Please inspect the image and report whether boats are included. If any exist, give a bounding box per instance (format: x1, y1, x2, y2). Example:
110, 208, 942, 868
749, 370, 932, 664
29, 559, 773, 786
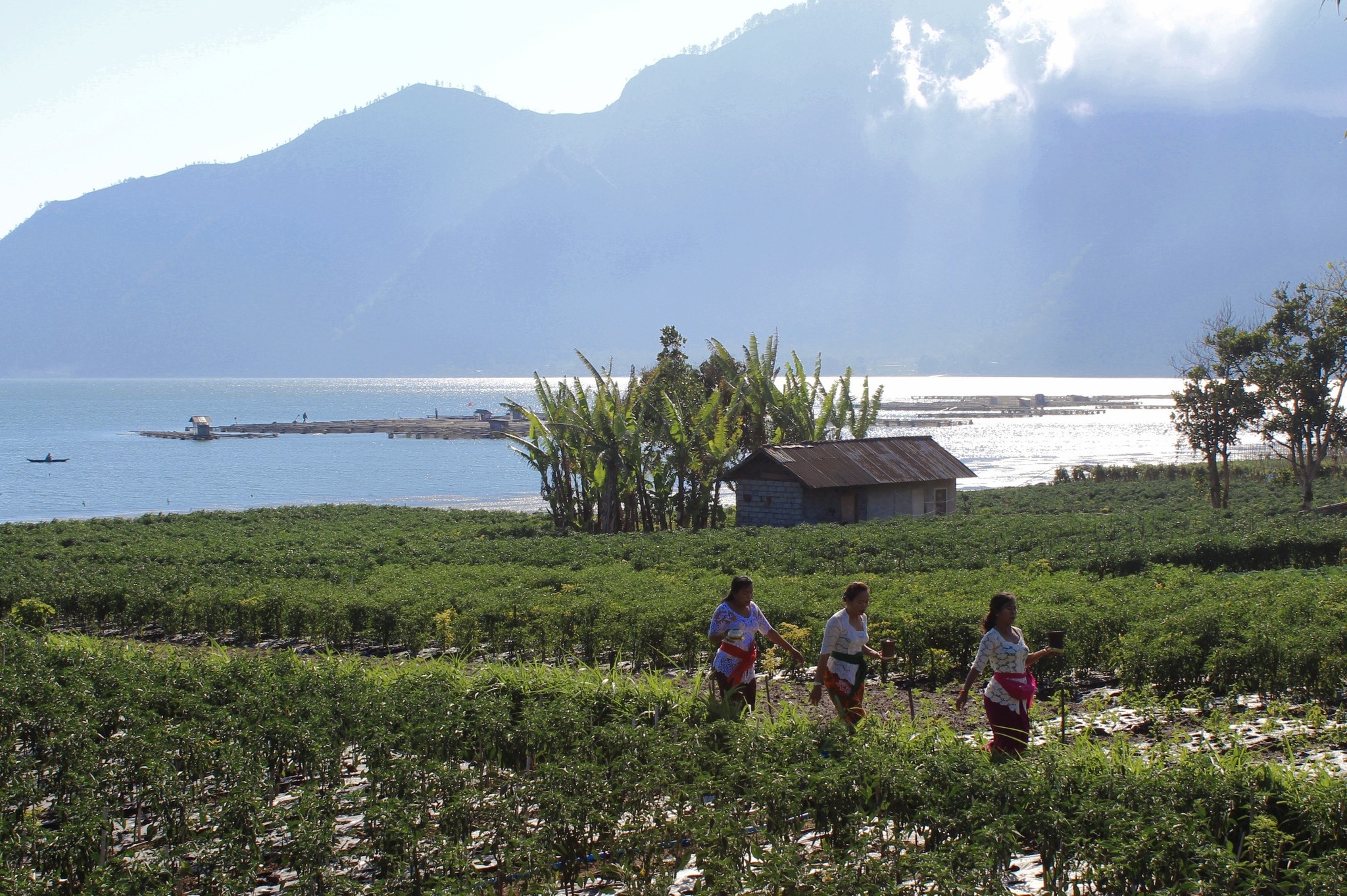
26, 458, 71, 462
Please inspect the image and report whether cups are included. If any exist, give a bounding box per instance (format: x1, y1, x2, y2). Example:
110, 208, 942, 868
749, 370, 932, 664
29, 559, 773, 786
880, 639, 897, 657
1046, 631, 1064, 649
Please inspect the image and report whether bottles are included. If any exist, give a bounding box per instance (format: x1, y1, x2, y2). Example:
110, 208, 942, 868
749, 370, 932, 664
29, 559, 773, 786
728, 628, 744, 640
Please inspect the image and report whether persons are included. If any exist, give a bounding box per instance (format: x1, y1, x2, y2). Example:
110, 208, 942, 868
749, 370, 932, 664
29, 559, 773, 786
303, 412, 308, 422
434, 408, 438, 418
45, 453, 54, 460
955, 592, 1057, 754
709, 575, 805, 713
808, 582, 893, 726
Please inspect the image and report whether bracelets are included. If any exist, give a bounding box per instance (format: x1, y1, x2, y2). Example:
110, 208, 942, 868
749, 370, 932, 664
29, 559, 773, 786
814, 682, 822, 686
959, 690, 968, 694
